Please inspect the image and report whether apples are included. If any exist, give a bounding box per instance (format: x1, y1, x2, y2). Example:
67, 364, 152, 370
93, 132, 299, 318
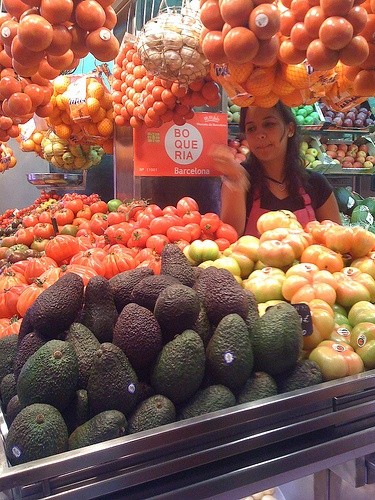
227, 138, 250, 163
320, 102, 375, 127
225, 95, 320, 125
296, 134, 323, 169
321, 142, 375, 168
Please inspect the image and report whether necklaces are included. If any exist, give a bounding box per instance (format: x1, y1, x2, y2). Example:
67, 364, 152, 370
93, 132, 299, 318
264, 174, 289, 192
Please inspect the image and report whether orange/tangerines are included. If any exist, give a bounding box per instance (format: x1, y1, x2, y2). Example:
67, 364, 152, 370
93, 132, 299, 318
0, 0, 120, 174
198, 0, 375, 98
112, 42, 221, 128
209, 58, 368, 112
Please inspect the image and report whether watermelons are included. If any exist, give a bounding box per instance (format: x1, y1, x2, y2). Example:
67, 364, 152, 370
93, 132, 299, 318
333, 186, 375, 234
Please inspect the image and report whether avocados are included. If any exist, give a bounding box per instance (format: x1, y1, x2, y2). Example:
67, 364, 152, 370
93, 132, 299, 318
0, 245, 323, 468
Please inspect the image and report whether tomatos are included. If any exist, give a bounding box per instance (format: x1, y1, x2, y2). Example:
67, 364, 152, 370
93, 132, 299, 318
0, 194, 238, 337
185, 211, 375, 379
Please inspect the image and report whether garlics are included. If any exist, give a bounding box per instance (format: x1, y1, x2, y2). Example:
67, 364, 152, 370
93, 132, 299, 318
136, 0, 209, 83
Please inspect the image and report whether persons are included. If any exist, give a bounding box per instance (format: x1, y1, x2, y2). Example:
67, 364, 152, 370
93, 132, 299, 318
207, 100, 343, 241
